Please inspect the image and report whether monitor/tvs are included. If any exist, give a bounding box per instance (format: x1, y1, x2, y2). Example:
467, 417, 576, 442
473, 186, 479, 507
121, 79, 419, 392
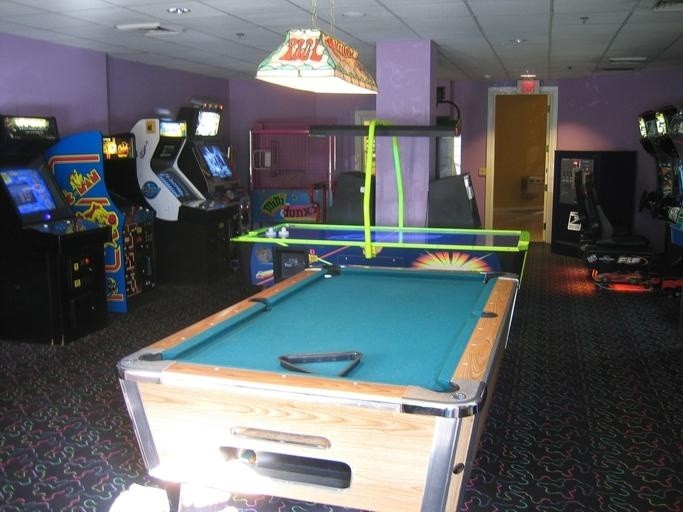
659, 161, 674, 199
197, 143, 234, 179
2, 166, 57, 215
158, 172, 193, 202
674, 157, 683, 203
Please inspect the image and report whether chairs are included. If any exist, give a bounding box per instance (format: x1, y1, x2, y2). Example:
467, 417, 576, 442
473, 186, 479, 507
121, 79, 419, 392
582, 158, 653, 285
573, 158, 652, 247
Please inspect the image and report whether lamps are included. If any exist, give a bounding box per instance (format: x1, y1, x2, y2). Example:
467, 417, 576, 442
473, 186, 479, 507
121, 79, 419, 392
253, 1, 383, 97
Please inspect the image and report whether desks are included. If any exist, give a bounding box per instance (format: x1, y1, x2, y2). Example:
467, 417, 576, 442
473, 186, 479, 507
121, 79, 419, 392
113, 261, 521, 512
